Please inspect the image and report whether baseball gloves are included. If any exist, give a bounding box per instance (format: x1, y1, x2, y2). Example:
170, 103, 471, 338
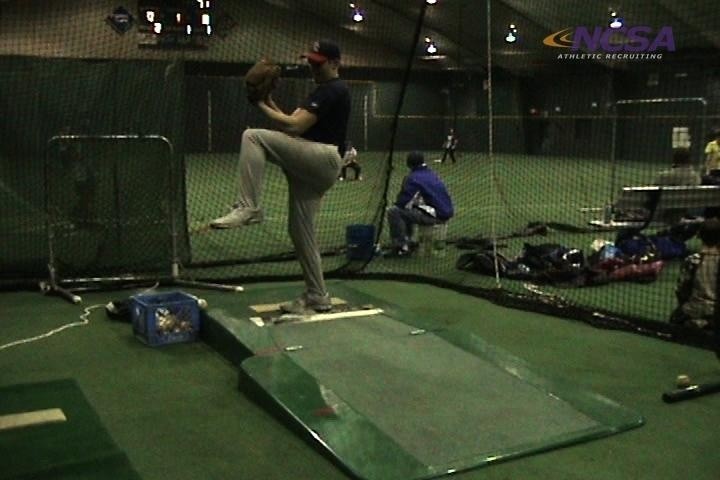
241, 57, 274, 104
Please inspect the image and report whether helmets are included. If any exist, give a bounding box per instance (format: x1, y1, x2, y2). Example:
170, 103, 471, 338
407, 151, 424, 166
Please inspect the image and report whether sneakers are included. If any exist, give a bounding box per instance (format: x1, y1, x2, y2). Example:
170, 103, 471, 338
208, 205, 264, 232
279, 292, 332, 313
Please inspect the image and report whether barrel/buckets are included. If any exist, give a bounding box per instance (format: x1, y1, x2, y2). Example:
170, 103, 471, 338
418, 222, 450, 260
345, 225, 374, 260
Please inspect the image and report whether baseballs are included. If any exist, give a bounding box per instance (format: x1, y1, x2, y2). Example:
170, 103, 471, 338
675, 374, 691, 388
196, 297, 209, 309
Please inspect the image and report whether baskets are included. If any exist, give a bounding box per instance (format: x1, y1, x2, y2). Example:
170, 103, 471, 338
128, 290, 200, 347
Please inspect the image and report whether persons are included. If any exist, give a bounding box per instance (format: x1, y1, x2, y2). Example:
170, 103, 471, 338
674, 218, 720, 328
653, 146, 703, 188
705, 126, 720, 185
208, 36, 352, 312
383, 151, 454, 258
440, 127, 459, 165
336, 140, 363, 181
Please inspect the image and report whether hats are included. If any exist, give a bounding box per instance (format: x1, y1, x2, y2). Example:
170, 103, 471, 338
299, 40, 341, 63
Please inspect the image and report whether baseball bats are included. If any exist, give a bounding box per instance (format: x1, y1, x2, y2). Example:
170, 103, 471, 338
154, 306, 193, 342
662, 381, 720, 402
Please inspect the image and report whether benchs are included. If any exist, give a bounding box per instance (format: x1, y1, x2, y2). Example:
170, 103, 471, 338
576, 183, 720, 241
